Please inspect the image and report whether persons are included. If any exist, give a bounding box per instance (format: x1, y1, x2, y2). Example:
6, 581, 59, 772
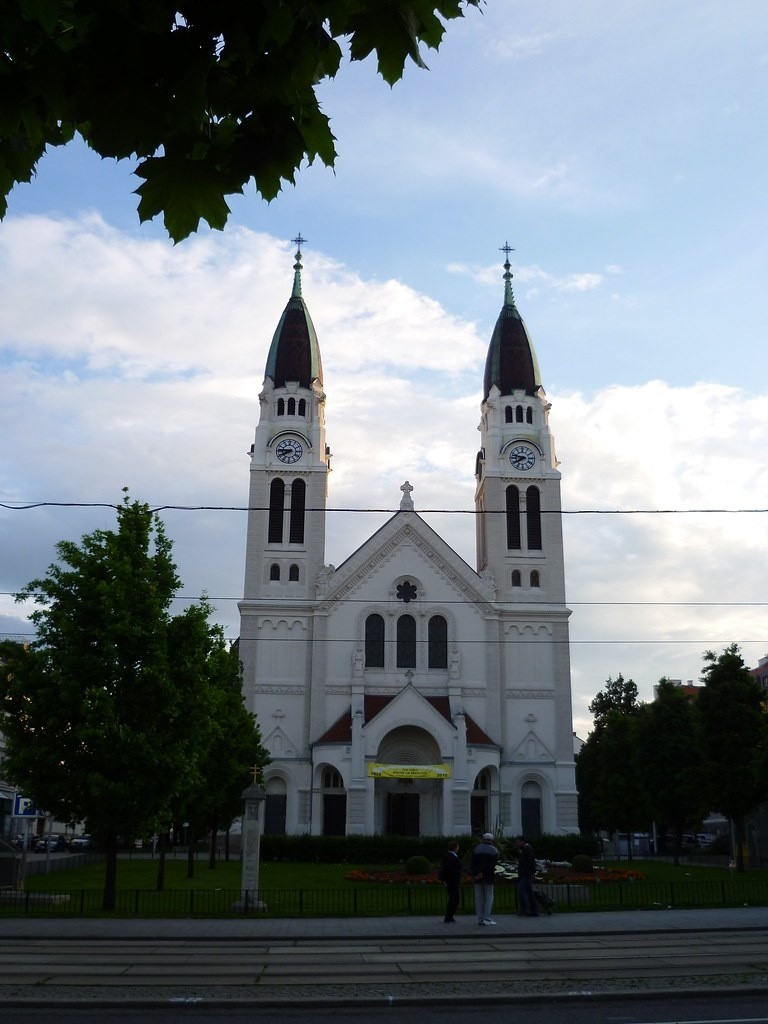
514, 836, 539, 917
440, 841, 463, 924
469, 833, 498, 925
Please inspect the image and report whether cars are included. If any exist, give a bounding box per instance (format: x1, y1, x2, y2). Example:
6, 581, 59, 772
35, 835, 66, 853
650, 834, 707, 855
69, 834, 93, 851
64, 834, 78, 846
12, 833, 39, 848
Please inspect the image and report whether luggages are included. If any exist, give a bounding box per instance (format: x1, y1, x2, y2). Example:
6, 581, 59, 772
533, 888, 556, 914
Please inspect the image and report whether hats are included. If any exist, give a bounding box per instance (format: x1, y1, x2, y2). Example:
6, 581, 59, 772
484, 832, 493, 839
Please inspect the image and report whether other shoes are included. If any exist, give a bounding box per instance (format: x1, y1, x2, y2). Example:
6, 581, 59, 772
517, 912, 527, 915
443, 918, 456, 924
479, 920, 484, 926
483, 919, 497, 925
526, 912, 540, 917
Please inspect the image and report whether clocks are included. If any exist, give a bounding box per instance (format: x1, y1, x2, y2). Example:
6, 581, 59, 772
508, 446, 535, 471
276, 439, 303, 465
